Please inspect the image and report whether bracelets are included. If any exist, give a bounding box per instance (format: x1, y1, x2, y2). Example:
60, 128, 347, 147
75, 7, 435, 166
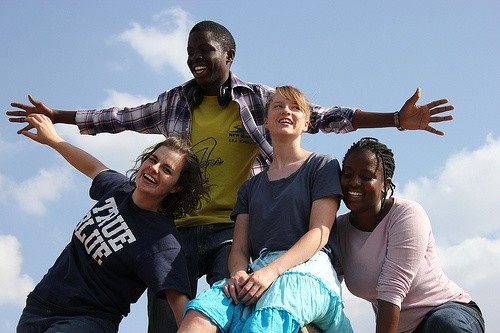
394, 111, 405, 132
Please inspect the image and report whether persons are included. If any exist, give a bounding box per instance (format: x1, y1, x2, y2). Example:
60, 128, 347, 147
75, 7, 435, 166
16, 114, 204, 333
175, 86, 353, 333
327, 137, 485, 333
5, 20, 455, 333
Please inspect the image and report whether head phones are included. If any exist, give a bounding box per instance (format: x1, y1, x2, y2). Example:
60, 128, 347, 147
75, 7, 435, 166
186, 70, 232, 107
